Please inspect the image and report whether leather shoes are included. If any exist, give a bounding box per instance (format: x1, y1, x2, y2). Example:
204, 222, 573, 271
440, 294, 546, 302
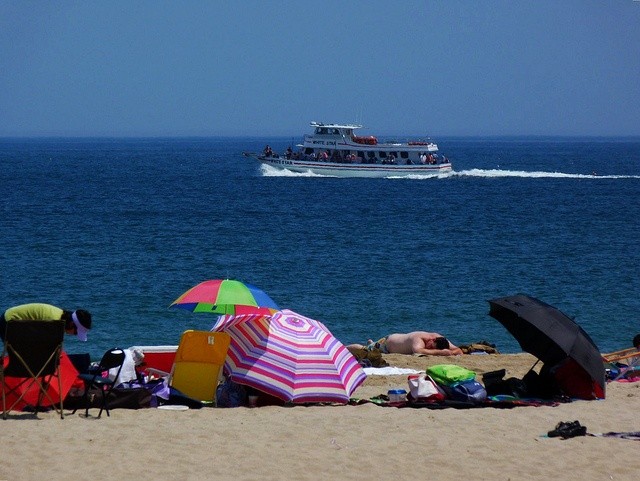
547, 421, 581, 438
556, 424, 587, 438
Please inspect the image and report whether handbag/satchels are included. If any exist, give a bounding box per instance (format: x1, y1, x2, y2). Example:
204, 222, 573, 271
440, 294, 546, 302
215, 376, 249, 407
407, 372, 444, 402
67, 387, 151, 410
119, 376, 170, 408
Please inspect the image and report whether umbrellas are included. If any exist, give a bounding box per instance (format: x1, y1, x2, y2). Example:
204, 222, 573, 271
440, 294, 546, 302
486, 291, 607, 402
167, 268, 283, 321
209, 309, 368, 405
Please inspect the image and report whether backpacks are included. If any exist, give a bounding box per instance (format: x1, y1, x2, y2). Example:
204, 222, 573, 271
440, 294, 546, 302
438, 377, 487, 403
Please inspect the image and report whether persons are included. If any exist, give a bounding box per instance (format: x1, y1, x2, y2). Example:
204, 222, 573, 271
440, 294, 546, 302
258, 143, 278, 163
286, 145, 329, 162
0, 302, 95, 345
416, 152, 447, 164
344, 152, 356, 164
384, 155, 395, 163
342, 330, 464, 358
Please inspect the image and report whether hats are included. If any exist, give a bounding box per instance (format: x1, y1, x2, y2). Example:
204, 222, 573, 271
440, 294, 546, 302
72, 308, 91, 341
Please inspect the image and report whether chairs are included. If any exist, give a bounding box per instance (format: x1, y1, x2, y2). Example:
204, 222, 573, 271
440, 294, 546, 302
146, 329, 231, 407
1, 320, 66, 419
71, 346, 125, 419
67, 353, 91, 374
136, 352, 175, 377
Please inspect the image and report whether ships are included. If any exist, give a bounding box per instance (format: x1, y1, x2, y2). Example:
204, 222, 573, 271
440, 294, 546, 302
243, 121, 452, 178
